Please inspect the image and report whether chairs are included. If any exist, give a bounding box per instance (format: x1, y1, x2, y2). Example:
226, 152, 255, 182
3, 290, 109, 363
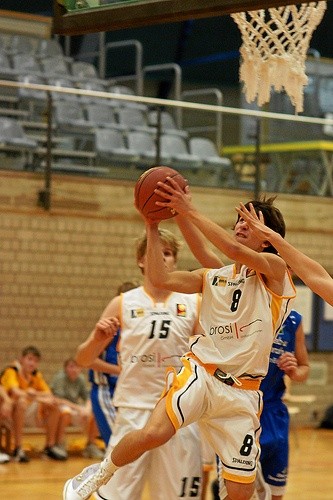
0, 32, 231, 186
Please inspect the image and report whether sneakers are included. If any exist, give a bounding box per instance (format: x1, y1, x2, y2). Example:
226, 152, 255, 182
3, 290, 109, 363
63, 464, 113, 500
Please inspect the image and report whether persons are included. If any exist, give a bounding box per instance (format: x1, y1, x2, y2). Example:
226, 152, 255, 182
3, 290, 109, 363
0, 345, 107, 464
63, 176, 296, 500
236, 202, 333, 307
216, 309, 311, 500
73, 227, 216, 500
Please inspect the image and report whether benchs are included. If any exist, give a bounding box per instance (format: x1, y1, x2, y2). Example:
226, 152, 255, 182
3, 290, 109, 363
1, 423, 107, 455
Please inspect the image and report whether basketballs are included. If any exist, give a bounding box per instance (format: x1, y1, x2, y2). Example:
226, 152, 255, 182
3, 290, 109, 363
134, 166, 188, 221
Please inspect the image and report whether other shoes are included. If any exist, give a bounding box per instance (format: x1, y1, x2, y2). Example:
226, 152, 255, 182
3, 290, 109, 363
44, 446, 67, 461
83, 445, 103, 459
0, 452, 10, 463
14, 449, 28, 461
